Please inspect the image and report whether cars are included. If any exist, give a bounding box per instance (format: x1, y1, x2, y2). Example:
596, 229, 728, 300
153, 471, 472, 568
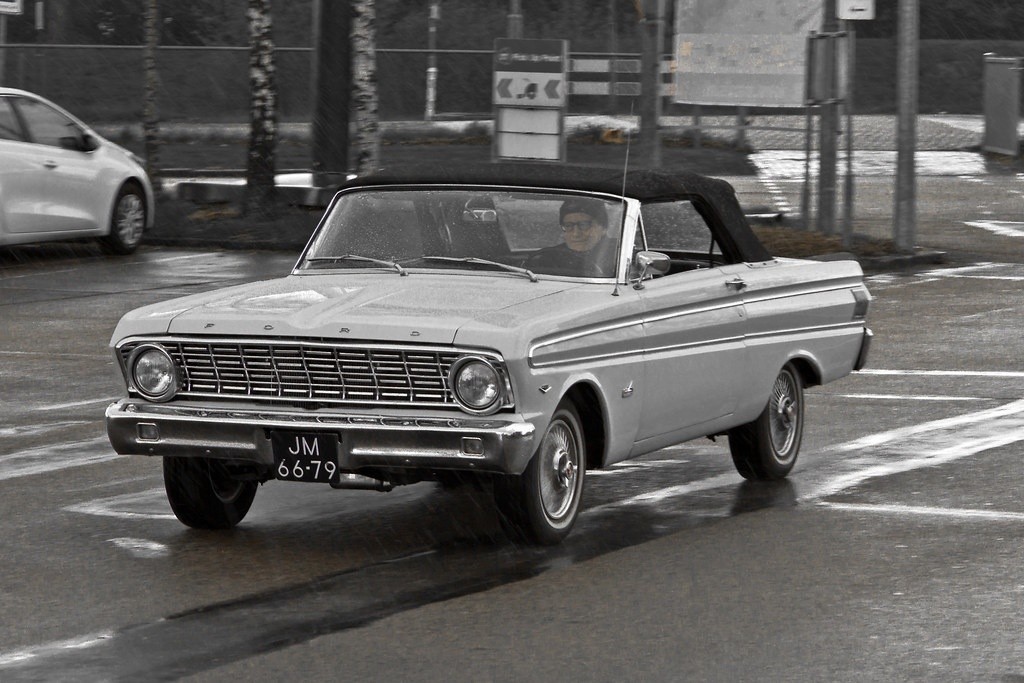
100, 155, 875, 549
0, 85, 157, 256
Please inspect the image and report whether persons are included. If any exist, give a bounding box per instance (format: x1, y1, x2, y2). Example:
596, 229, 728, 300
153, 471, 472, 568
519, 198, 618, 277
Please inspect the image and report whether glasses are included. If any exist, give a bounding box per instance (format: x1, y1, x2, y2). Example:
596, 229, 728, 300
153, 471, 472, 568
559, 221, 595, 233
